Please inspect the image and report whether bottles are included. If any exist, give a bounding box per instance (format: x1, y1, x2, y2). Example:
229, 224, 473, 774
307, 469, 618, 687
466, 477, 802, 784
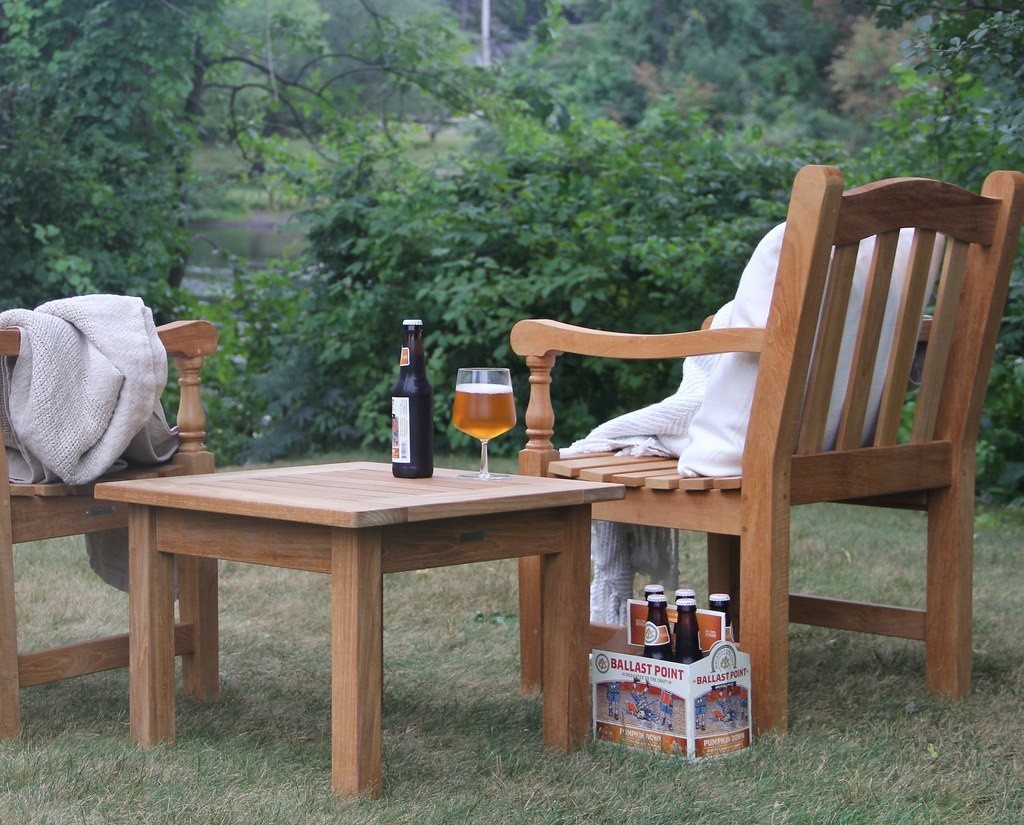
710, 593, 735, 643
392, 319, 434, 479
644, 584, 664, 603
674, 590, 696, 607
641, 594, 673, 661
672, 599, 703, 665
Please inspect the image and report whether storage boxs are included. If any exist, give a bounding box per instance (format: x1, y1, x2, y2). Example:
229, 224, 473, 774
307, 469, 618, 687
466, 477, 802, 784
590, 598, 753, 763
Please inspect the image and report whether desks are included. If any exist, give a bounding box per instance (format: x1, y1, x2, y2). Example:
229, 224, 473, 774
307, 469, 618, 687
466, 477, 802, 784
94, 460, 626, 798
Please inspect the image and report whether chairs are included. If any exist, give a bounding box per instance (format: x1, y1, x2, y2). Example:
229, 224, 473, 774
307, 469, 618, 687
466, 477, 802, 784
509, 164, 1024, 739
0, 293, 220, 737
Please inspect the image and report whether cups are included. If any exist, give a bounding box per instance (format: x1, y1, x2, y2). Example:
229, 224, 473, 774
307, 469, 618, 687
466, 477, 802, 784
452, 368, 516, 480
